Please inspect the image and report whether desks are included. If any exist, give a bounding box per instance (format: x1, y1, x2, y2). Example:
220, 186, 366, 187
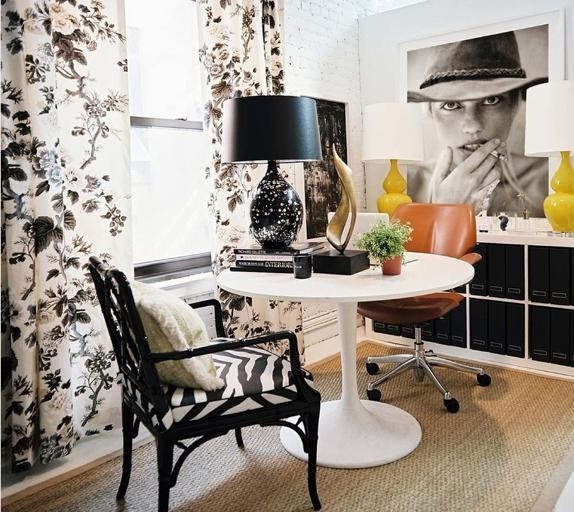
215, 252, 476, 470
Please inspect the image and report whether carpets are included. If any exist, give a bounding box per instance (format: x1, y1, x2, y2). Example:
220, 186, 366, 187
1, 340, 574, 512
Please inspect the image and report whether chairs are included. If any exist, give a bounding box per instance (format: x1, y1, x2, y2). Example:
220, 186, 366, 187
88, 256, 322, 511
356, 203, 491, 413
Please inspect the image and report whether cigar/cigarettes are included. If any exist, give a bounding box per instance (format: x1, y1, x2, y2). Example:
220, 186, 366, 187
477, 143, 510, 163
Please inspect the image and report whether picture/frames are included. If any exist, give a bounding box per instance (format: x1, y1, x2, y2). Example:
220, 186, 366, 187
295, 93, 349, 242
396, 9, 566, 231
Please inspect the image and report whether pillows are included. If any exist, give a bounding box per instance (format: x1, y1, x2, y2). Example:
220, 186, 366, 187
128, 277, 227, 393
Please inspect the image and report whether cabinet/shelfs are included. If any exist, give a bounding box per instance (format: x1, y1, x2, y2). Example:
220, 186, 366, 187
364, 231, 574, 384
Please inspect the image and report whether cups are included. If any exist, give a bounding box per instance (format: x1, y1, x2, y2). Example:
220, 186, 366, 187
292, 253, 311, 280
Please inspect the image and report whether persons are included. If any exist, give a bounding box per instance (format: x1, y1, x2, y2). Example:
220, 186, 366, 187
404, 32, 549, 219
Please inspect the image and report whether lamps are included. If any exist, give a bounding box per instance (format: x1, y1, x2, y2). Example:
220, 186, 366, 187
221, 95, 324, 253
524, 81, 574, 233
362, 101, 425, 221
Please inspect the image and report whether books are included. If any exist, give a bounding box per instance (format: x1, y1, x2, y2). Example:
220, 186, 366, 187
228, 241, 329, 274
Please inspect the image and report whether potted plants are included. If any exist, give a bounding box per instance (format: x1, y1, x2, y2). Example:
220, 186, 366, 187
353, 219, 414, 275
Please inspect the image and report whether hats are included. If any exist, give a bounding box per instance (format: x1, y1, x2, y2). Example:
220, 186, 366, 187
408, 31, 548, 108
